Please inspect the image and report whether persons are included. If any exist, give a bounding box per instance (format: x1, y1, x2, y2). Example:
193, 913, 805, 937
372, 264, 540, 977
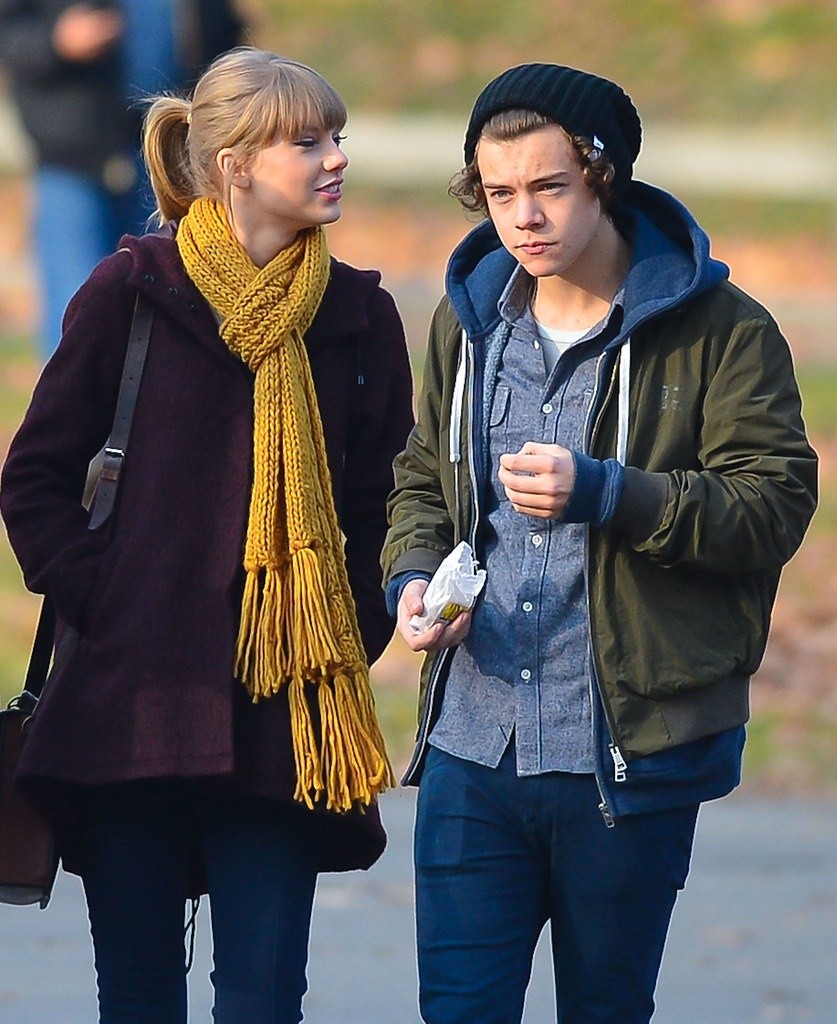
1, 0, 254, 367
378, 64, 818, 1024
0, 52, 412, 1024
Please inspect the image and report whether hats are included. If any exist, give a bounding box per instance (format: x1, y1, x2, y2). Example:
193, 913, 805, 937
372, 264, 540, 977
464, 62, 641, 194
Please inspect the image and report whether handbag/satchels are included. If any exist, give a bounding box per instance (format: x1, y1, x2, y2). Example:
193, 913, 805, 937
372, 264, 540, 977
0, 690, 61, 911
